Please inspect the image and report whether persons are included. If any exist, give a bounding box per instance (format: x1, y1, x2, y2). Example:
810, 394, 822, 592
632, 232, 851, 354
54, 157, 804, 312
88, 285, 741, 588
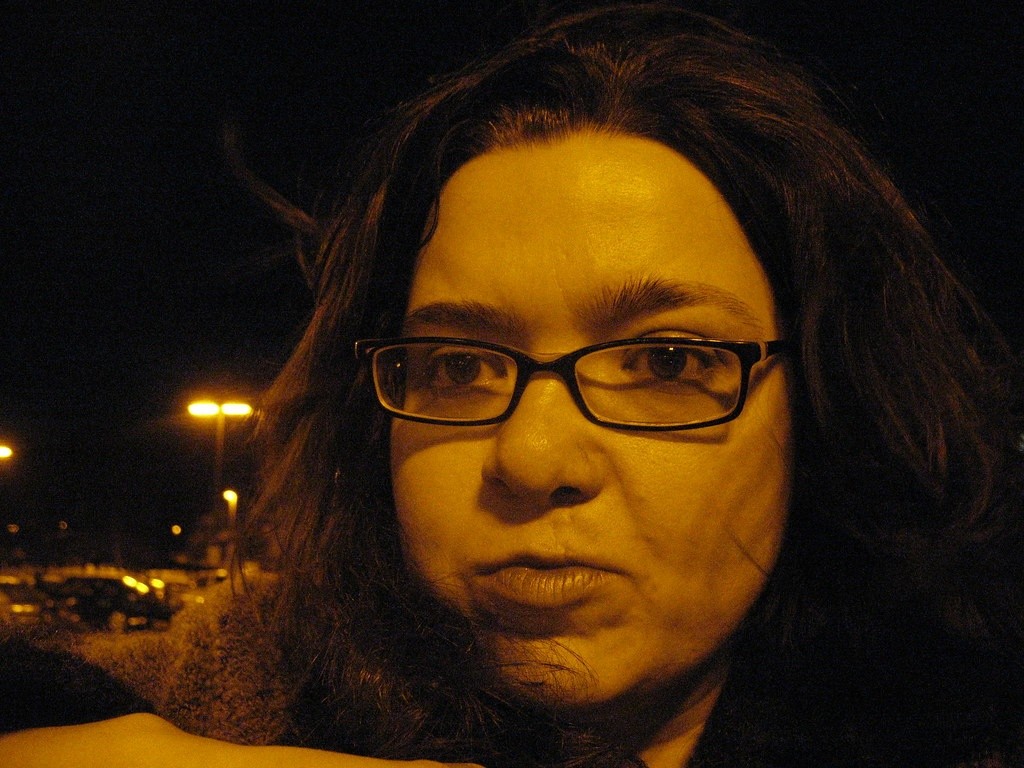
0, 1, 1024, 768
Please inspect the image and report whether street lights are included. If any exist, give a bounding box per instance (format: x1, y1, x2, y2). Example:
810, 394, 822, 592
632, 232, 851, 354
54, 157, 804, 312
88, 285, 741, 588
185, 401, 253, 520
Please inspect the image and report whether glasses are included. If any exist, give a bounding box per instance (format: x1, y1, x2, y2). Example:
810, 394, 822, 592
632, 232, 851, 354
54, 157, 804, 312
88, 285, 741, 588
355, 338, 791, 431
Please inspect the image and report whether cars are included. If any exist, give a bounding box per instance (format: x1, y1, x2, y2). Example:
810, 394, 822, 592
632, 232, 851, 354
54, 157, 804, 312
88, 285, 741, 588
35, 569, 185, 633
